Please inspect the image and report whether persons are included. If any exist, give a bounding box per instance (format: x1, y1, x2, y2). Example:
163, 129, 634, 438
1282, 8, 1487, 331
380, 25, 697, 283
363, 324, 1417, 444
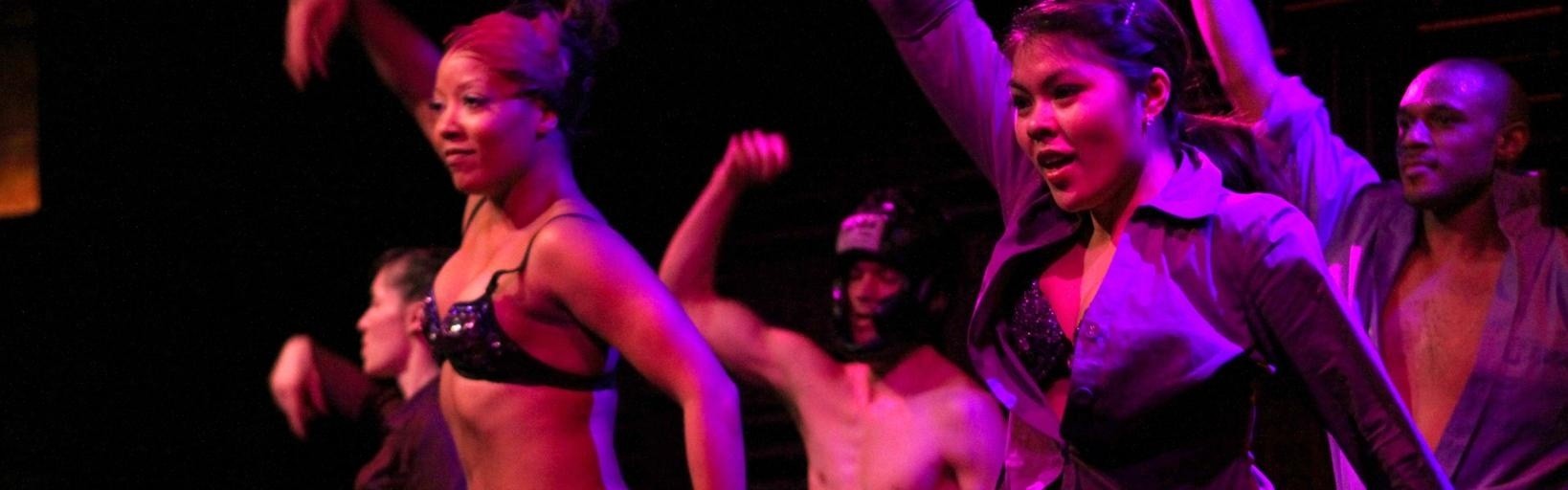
655, 126, 1006, 490
1189, 0, 1567, 488
1175, 58, 1261, 193
866, 2, 1459, 490
281, 0, 751, 490
266, 244, 471, 490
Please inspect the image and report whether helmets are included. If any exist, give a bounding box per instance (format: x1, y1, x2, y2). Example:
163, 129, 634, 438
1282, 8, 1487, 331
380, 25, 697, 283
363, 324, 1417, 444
829, 206, 955, 341
